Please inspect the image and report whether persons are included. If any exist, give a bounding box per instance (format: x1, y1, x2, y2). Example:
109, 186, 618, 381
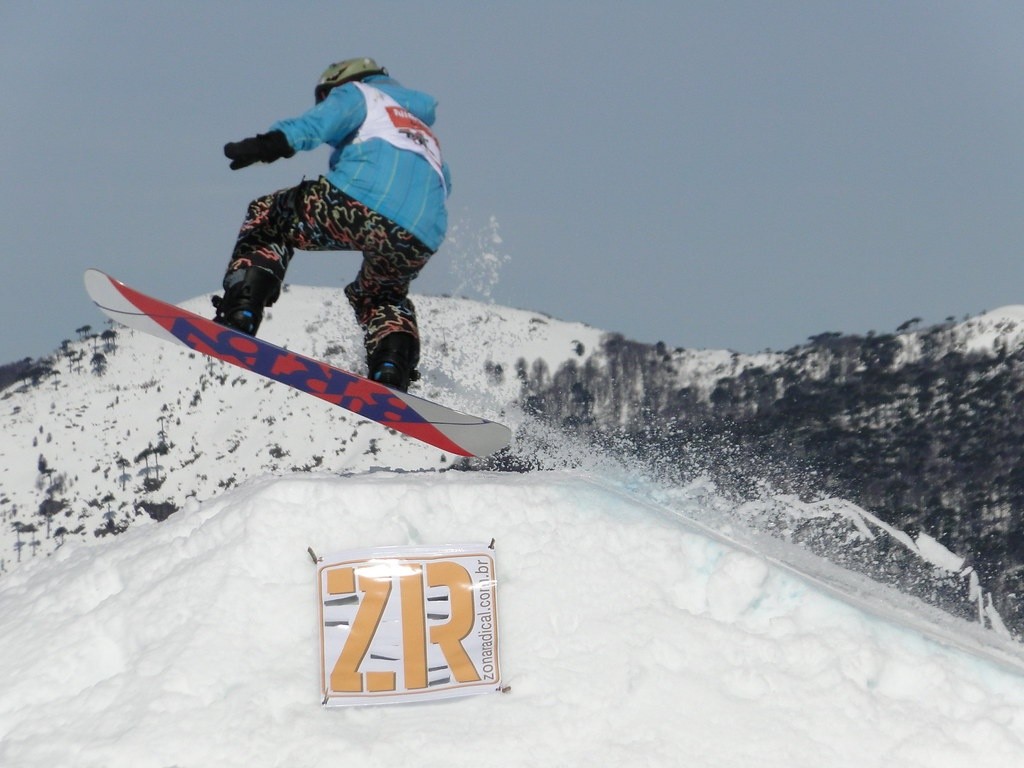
198, 55, 451, 393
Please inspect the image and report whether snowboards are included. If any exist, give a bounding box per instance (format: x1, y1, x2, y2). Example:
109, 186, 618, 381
81, 267, 514, 461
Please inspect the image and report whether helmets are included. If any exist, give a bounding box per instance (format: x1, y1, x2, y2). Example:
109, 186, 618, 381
315, 57, 382, 107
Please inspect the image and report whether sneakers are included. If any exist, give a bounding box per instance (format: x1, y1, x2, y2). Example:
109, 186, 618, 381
213, 284, 263, 334
366, 350, 421, 396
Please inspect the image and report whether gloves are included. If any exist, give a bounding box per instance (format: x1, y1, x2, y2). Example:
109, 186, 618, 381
224, 131, 295, 169
